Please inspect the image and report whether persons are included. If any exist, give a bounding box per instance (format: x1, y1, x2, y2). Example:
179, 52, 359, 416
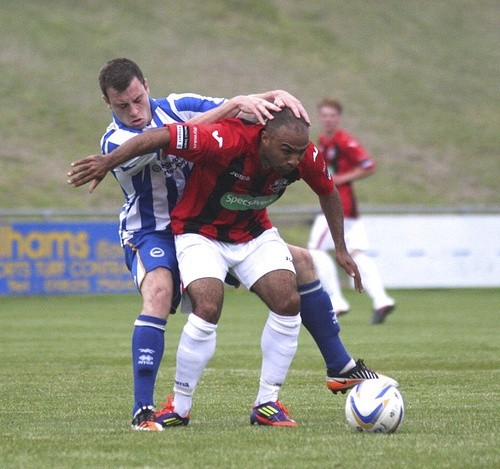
96, 57, 400, 430
67, 104, 364, 426
307, 99, 395, 324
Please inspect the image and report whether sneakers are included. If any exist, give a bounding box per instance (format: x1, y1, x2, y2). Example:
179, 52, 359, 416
130, 401, 164, 430
251, 400, 297, 427
152, 396, 189, 426
326, 358, 399, 394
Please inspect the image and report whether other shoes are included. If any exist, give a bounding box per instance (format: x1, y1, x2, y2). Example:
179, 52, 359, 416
371, 305, 395, 323
332, 303, 349, 315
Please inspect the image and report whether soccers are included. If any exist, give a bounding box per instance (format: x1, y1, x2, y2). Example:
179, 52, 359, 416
345, 378, 404, 434
